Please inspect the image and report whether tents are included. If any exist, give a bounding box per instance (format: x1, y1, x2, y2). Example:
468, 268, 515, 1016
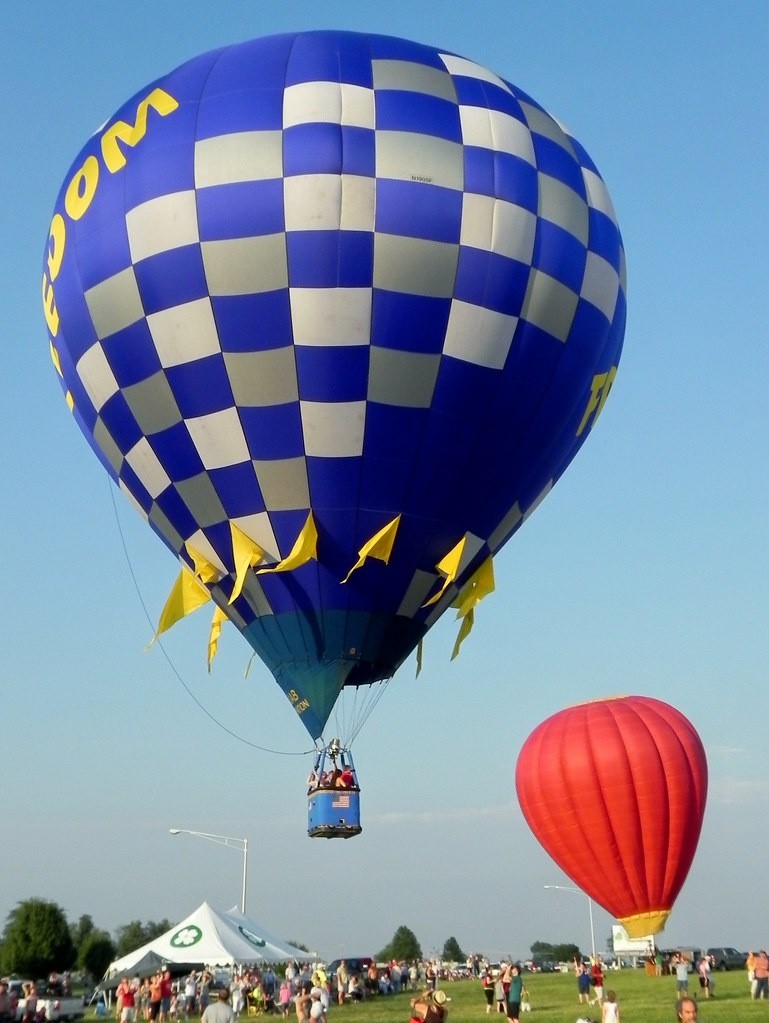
86, 902, 318, 1010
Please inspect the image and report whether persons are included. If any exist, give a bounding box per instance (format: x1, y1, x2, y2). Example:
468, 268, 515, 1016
576, 956, 607, 1007
602, 990, 619, 1023
482, 954, 531, 1023
698, 955, 715, 997
466, 956, 490, 979
676, 997, 697, 1023
116, 961, 330, 1023
746, 950, 769, 999
0, 972, 72, 1023
654, 952, 662, 976
307, 764, 355, 788
672, 956, 689, 999
337, 959, 454, 1005
409, 991, 448, 1023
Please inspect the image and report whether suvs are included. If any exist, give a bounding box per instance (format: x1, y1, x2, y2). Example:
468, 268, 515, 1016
705, 946, 748, 971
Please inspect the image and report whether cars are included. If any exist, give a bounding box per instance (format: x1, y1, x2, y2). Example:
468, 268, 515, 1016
489, 963, 503, 976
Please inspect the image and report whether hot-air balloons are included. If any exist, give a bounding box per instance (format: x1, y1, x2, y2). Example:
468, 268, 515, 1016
511, 693, 710, 978
36, 27, 629, 843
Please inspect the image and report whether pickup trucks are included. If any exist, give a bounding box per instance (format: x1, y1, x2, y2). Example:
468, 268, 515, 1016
7, 978, 86, 1023
326, 955, 391, 991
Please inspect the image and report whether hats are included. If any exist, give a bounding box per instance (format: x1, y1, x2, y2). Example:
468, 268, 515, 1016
310, 1001, 323, 1019
432, 990, 446, 1008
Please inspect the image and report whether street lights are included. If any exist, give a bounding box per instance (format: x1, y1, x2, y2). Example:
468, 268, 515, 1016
543, 884, 596, 963
169, 828, 248, 915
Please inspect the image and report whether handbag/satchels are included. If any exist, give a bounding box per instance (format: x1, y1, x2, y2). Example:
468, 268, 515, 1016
590, 977, 597, 986
